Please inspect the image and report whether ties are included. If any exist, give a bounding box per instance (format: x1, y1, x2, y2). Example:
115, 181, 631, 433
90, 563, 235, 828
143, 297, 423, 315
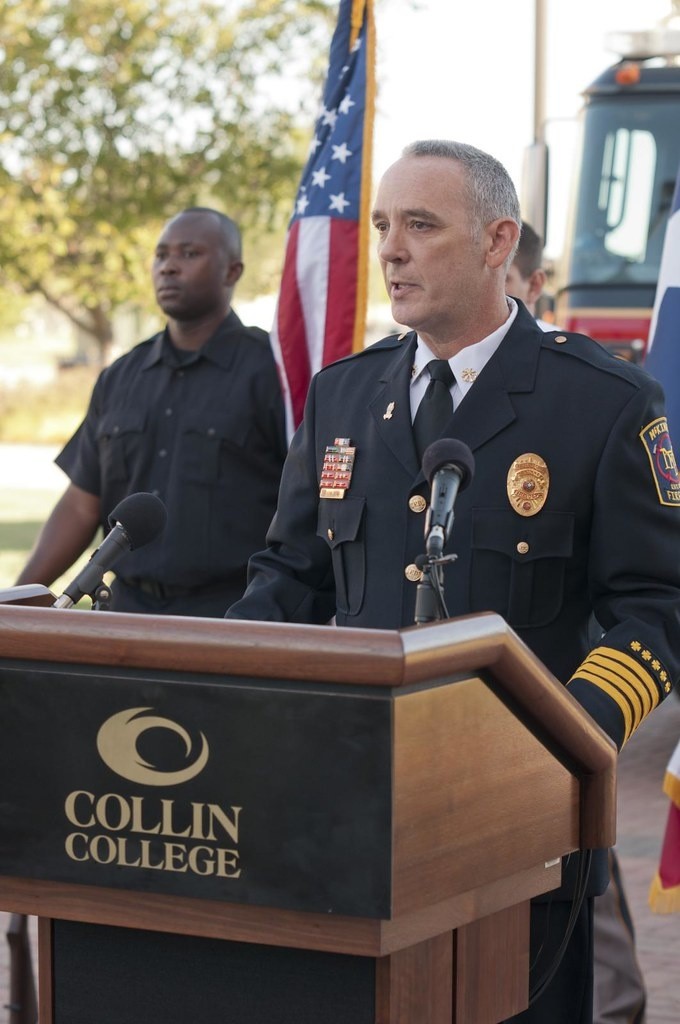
412, 360, 457, 467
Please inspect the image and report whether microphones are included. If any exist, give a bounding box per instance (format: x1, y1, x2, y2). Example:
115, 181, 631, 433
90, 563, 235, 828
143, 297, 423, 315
51, 492, 168, 608
421, 439, 477, 569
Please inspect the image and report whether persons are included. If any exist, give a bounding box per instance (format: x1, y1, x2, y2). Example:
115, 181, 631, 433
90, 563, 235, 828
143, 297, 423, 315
219, 140, 680, 1024
11, 201, 295, 623
502, 219, 562, 338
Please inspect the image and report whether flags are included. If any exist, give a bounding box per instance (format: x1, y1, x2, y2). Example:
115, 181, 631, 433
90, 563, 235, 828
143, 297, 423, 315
635, 153, 680, 919
257, 0, 383, 463
